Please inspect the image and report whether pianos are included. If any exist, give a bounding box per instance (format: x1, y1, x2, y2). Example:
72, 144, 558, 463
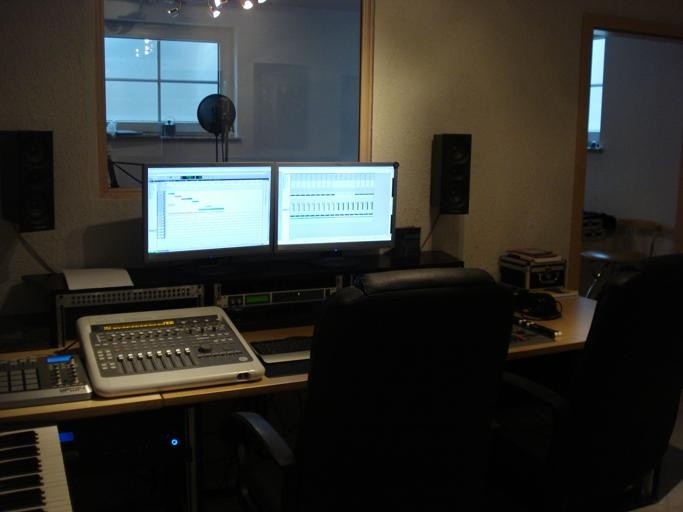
0, 425, 73, 512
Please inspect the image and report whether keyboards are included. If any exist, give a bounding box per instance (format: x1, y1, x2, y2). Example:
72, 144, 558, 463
248, 336, 313, 363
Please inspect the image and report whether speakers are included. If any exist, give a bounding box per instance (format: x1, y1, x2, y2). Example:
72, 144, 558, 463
428, 131, 472, 216
0, 130, 57, 232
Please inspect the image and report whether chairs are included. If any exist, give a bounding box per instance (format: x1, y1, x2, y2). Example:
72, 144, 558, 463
581, 218, 665, 297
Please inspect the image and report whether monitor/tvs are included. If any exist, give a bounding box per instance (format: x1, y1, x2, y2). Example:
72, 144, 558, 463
273, 160, 399, 268
141, 162, 274, 276
